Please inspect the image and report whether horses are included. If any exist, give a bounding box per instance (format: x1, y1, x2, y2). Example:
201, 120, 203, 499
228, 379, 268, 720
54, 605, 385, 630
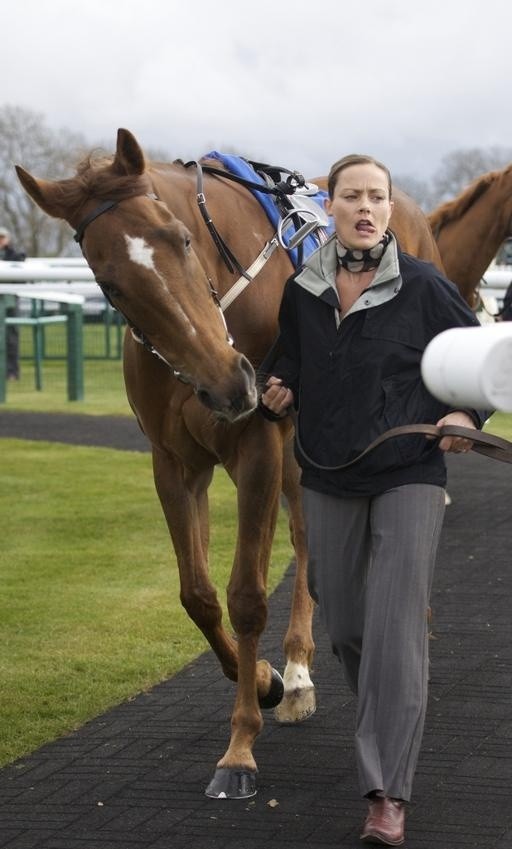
426, 159, 512, 300
13, 123, 451, 806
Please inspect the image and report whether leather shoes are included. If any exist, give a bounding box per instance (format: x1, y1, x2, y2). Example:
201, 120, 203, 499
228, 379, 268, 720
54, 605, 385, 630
360, 797, 406, 846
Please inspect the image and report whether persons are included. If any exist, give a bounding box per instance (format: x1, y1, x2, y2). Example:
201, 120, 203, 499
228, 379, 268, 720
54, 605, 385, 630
0, 229, 26, 381
263, 155, 498, 847
